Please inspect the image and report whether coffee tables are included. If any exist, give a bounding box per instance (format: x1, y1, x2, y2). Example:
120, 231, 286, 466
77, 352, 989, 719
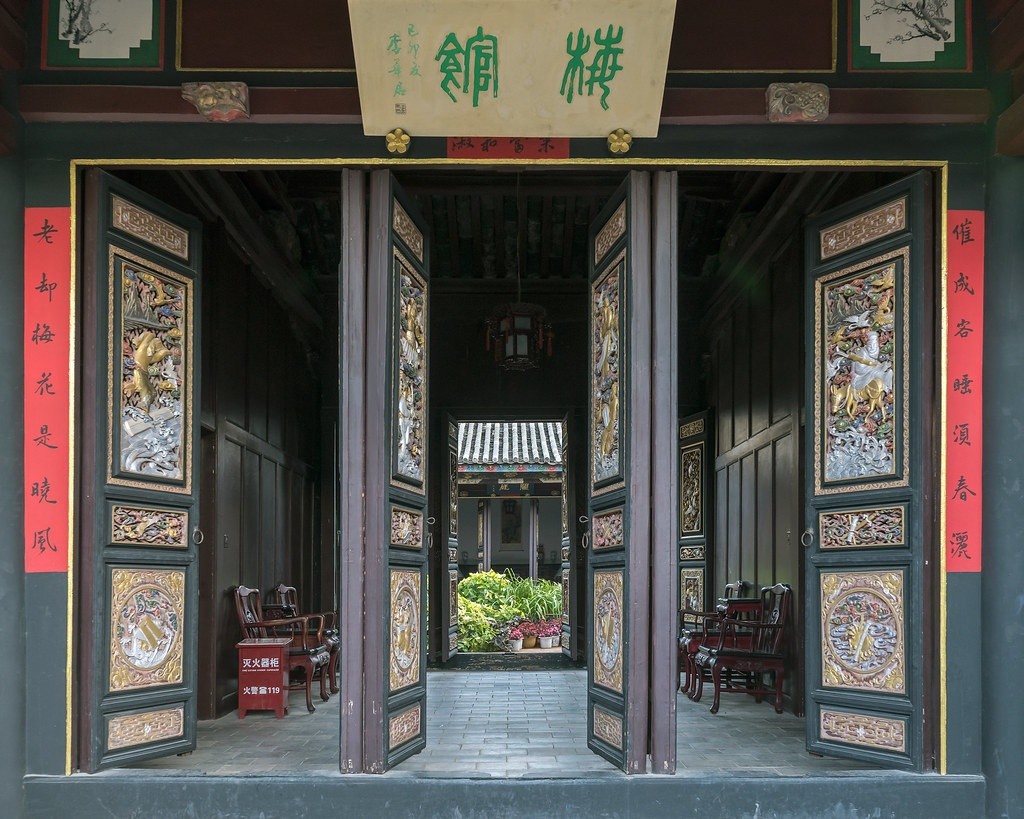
267, 629, 339, 651
681, 628, 759, 700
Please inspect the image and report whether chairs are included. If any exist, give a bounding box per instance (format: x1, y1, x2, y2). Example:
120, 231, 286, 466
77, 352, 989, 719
679, 578, 793, 714
233, 586, 340, 715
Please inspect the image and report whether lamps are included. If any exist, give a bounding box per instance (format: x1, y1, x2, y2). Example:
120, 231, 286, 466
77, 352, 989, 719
486, 173, 553, 370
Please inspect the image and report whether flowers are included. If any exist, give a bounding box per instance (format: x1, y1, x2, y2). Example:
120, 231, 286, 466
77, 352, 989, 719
507, 619, 563, 639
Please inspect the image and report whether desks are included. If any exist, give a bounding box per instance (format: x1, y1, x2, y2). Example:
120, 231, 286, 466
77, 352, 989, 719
716, 597, 764, 629
262, 603, 296, 629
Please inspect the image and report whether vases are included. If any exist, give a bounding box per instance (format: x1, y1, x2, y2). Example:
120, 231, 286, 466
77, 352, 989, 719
508, 634, 561, 652
